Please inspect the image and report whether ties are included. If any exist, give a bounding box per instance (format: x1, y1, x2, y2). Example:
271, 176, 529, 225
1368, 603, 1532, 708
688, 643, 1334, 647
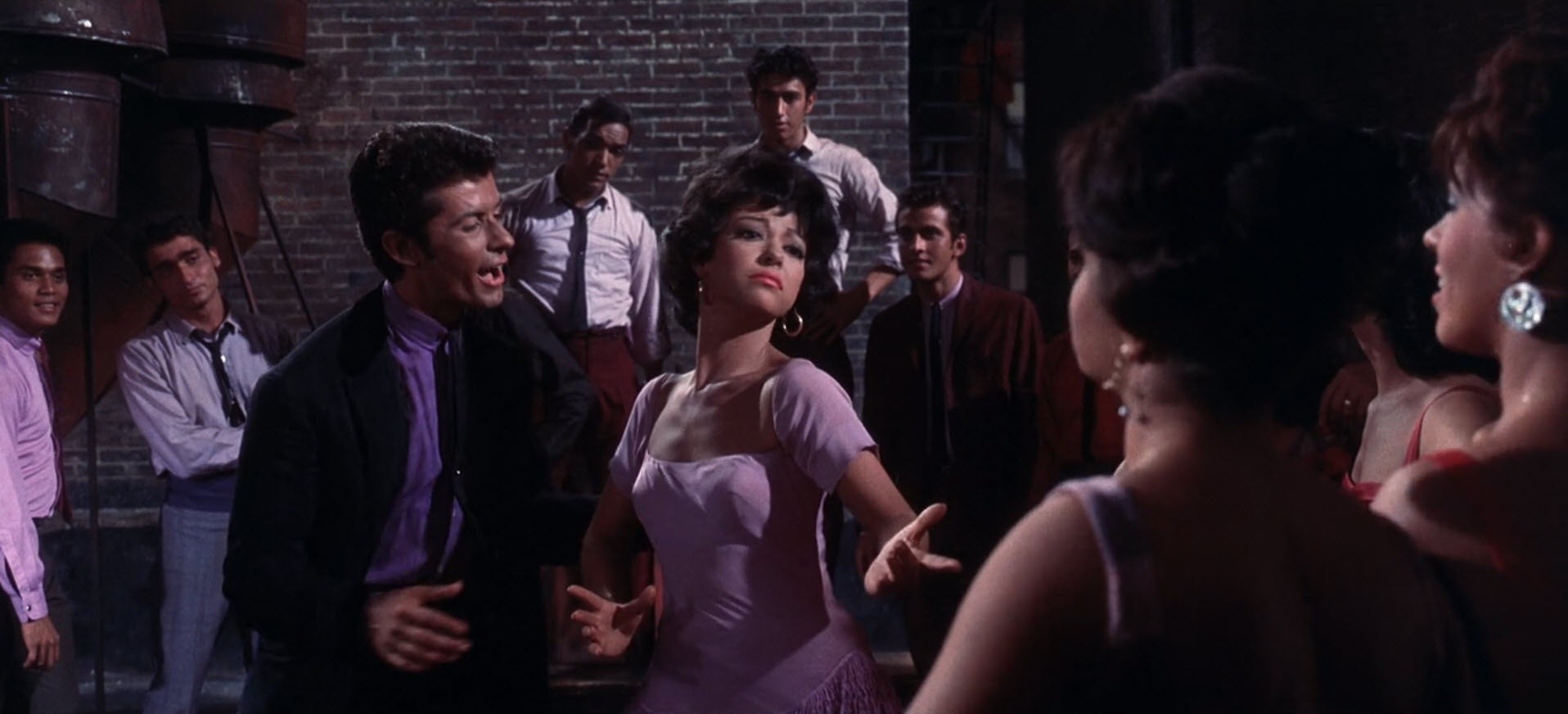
930, 303, 942, 469
39, 343, 74, 524
559, 201, 603, 332
191, 326, 247, 426
423, 332, 462, 584
1081, 380, 1097, 479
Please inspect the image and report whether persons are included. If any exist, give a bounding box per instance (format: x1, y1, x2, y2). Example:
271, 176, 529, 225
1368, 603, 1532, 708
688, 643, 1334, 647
1030, 227, 1124, 504
659, 43, 905, 578
0, 217, 73, 714
1368, 20, 1568, 714
905, 65, 1480, 714
861, 182, 1045, 667
115, 211, 293, 714
566, 145, 961, 714
500, 97, 671, 671
221, 121, 579, 714
499, 289, 590, 464
1338, 128, 1501, 509
